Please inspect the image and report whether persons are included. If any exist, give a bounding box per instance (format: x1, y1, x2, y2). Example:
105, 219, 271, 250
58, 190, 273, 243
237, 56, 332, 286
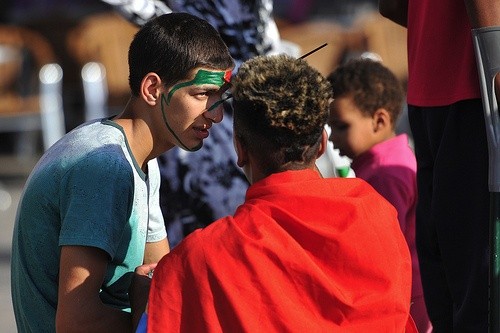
147, 53, 416, 333
11, 12, 236, 333
327, 59, 432, 332
379, 0, 500, 333
